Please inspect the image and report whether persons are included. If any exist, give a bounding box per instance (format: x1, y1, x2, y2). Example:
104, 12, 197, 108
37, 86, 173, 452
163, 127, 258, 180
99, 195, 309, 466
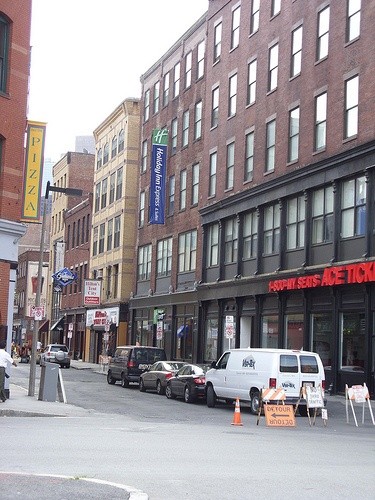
11, 339, 42, 359
0, 340, 18, 403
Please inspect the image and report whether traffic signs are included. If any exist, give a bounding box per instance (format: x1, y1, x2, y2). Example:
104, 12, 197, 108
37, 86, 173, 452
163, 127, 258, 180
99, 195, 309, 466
263, 404, 294, 428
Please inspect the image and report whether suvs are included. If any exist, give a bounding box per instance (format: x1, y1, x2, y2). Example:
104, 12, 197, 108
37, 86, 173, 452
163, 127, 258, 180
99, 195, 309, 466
40, 344, 69, 369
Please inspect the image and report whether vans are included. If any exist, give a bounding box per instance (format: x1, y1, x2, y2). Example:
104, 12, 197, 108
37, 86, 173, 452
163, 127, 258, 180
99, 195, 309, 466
206, 348, 326, 417
106, 346, 166, 388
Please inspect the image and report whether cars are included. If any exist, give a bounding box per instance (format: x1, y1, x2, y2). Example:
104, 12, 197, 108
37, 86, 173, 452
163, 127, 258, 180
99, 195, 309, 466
165, 364, 211, 404
138, 360, 186, 393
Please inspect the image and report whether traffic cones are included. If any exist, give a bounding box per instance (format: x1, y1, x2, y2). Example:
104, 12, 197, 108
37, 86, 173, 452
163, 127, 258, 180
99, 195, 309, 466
231, 397, 242, 425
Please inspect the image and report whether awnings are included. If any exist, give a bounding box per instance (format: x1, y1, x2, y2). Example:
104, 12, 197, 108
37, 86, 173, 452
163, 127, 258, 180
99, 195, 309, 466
178, 325, 191, 336
39, 315, 66, 332
